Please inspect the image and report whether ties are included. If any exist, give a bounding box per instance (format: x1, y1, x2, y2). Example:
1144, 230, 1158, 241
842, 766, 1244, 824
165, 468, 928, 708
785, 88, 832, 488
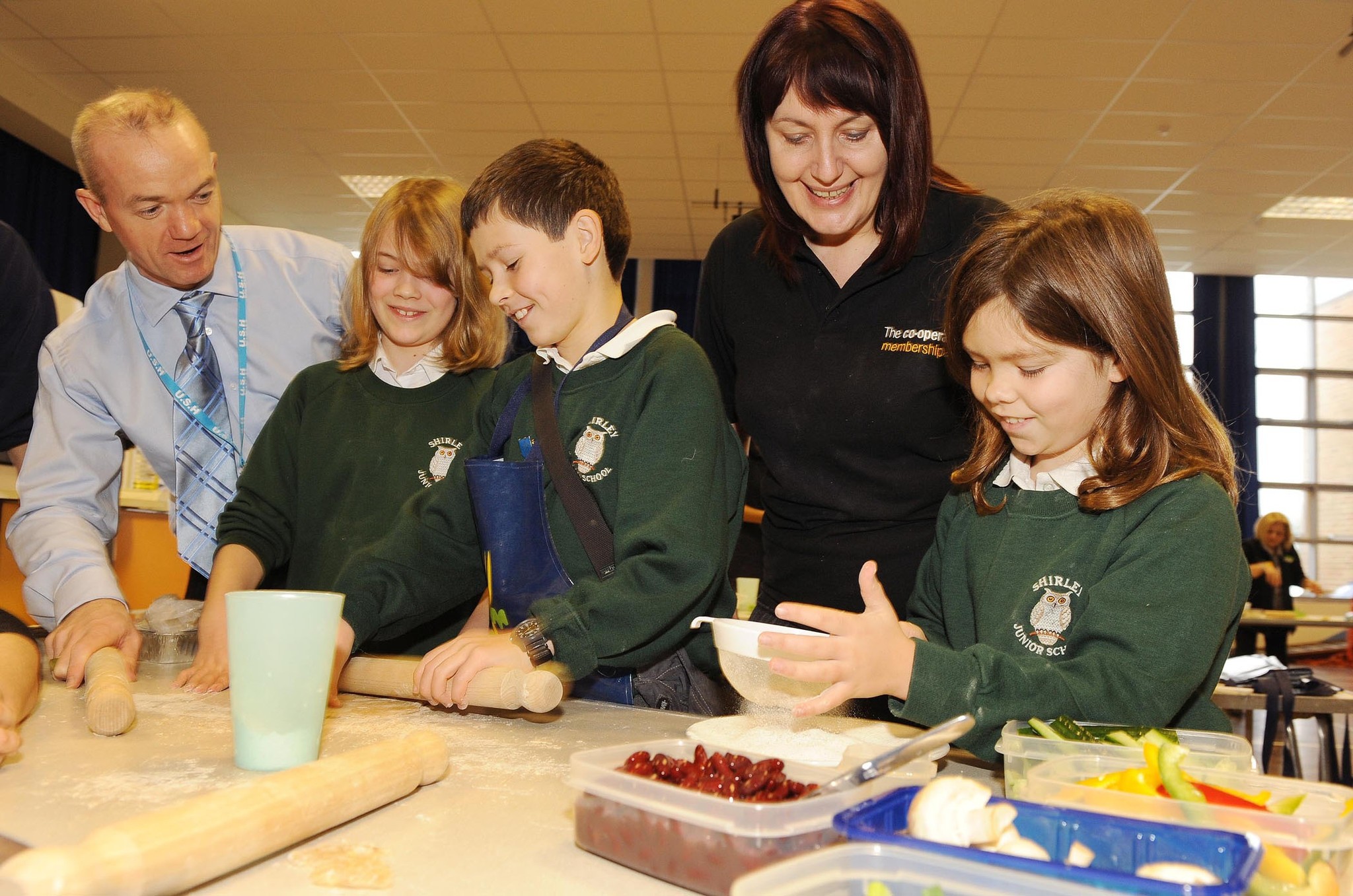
173, 290, 238, 580
1273, 555, 1283, 610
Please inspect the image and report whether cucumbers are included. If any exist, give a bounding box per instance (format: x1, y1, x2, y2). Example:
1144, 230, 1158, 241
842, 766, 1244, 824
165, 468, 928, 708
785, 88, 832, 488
1027, 715, 1180, 746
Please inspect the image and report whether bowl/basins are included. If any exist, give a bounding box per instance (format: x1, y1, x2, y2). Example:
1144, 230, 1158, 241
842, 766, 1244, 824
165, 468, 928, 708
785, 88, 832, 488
137, 628, 198, 664
689, 616, 834, 708
567, 713, 1352, 896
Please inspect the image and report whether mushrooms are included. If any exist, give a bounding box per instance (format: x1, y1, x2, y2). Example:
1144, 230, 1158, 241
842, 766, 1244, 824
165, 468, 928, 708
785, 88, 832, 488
895, 775, 1224, 885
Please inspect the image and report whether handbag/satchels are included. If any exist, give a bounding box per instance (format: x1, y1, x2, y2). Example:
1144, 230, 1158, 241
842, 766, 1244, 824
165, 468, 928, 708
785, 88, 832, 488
634, 665, 739, 717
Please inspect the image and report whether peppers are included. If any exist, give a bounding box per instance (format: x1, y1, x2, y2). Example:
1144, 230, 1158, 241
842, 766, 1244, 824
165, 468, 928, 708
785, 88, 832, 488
1080, 741, 1353, 895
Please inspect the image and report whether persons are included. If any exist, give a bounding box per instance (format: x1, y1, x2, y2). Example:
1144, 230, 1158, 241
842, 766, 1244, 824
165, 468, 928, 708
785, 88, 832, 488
1, 86, 750, 752
759, 188, 1251, 765
692, 0, 1017, 735
1240, 512, 1329, 666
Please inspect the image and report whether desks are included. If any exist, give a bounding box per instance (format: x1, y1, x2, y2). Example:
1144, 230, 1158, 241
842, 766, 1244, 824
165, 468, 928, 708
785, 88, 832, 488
1208, 676, 1353, 785
0, 446, 192, 627
1238, 608, 1353, 628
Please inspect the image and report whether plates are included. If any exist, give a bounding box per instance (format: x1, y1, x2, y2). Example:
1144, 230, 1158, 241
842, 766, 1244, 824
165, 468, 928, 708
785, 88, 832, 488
686, 714, 951, 762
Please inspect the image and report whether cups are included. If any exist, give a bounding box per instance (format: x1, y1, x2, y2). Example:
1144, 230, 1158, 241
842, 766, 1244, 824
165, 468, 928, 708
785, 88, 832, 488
225, 589, 346, 771
